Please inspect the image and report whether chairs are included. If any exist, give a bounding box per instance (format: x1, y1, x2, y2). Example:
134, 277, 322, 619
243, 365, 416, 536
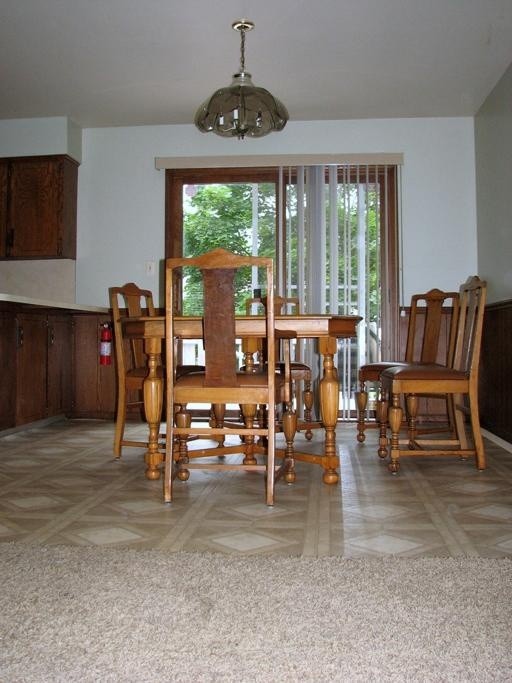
243, 295, 313, 440
165, 248, 284, 506
107, 283, 178, 459
379, 271, 488, 474
355, 287, 460, 443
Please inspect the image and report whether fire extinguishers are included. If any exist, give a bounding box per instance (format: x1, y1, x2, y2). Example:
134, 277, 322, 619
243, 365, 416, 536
99, 321, 112, 365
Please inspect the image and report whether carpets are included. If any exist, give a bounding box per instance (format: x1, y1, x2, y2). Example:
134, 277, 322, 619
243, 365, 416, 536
0, 543, 512, 681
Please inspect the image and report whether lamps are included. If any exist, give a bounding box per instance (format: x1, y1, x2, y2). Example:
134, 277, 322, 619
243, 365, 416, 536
193, 20, 288, 139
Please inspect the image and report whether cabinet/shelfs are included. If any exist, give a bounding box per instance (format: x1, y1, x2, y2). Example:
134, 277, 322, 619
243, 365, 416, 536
18, 295, 73, 431
73, 307, 167, 422
0, 293, 17, 438
0, 153, 81, 261
